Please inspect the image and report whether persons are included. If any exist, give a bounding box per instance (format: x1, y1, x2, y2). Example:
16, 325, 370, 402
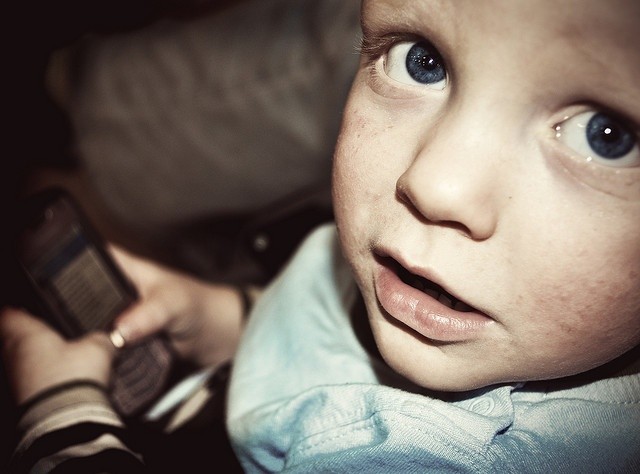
1, 0, 640, 473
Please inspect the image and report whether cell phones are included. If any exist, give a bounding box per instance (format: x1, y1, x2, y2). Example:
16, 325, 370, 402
7, 186, 182, 424
